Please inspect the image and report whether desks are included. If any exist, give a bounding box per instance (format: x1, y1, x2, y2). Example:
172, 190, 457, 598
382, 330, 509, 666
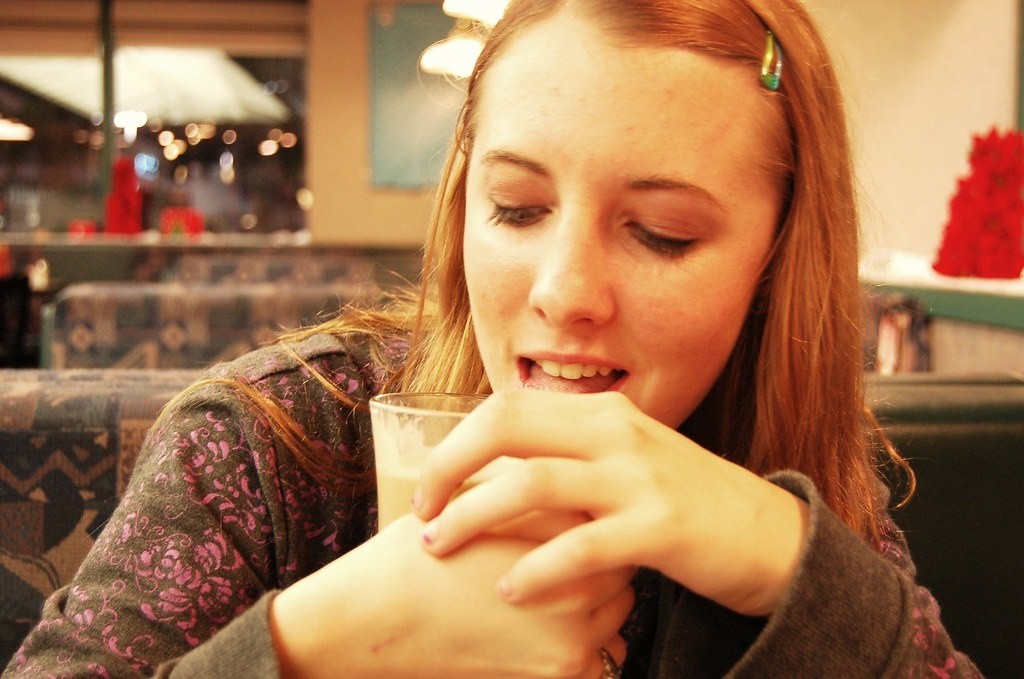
860, 277, 1024, 336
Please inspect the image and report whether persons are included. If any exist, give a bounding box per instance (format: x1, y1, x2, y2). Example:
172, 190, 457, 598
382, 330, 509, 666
0, 0, 983, 679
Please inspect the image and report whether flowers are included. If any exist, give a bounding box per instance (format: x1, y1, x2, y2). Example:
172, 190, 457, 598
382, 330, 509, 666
932, 129, 1024, 279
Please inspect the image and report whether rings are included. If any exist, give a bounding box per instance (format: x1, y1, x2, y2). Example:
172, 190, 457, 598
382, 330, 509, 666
596, 647, 621, 679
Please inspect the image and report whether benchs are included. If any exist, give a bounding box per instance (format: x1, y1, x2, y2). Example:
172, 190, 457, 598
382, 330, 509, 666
39, 281, 382, 368
134, 247, 422, 287
1, 369, 210, 672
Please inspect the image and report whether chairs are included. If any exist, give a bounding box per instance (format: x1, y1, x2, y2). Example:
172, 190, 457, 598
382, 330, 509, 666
660, 379, 1024, 678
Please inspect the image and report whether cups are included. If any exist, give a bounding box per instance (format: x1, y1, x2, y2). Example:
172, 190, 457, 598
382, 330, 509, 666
367, 392, 490, 534
